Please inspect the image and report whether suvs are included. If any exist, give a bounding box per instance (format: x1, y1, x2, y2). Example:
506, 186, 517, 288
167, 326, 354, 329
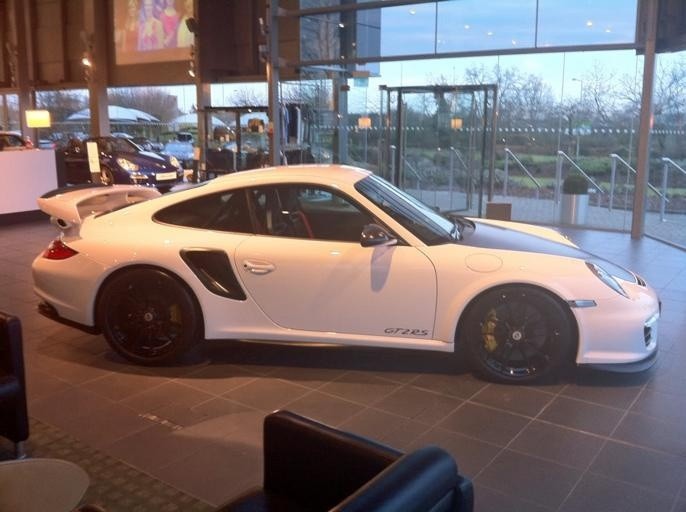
155, 131, 194, 168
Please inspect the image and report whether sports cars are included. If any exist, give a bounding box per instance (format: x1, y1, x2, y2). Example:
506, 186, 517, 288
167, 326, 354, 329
32, 163, 662, 385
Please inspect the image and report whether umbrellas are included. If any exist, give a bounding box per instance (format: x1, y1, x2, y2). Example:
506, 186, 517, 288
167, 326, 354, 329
165, 112, 228, 127
64, 105, 161, 122
227, 111, 270, 128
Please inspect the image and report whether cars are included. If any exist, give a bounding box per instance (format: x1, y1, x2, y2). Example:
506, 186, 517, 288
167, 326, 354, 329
37, 139, 54, 149
54, 135, 184, 192
0, 131, 39, 152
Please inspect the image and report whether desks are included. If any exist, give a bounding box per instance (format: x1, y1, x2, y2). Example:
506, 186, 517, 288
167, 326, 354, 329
0, 459, 88, 511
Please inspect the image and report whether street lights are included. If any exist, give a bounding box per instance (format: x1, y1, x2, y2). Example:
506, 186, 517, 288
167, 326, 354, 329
571, 78, 583, 158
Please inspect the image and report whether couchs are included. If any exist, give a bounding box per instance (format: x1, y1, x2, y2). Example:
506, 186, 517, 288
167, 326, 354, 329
214, 410, 473, 512
0, 311, 28, 460
